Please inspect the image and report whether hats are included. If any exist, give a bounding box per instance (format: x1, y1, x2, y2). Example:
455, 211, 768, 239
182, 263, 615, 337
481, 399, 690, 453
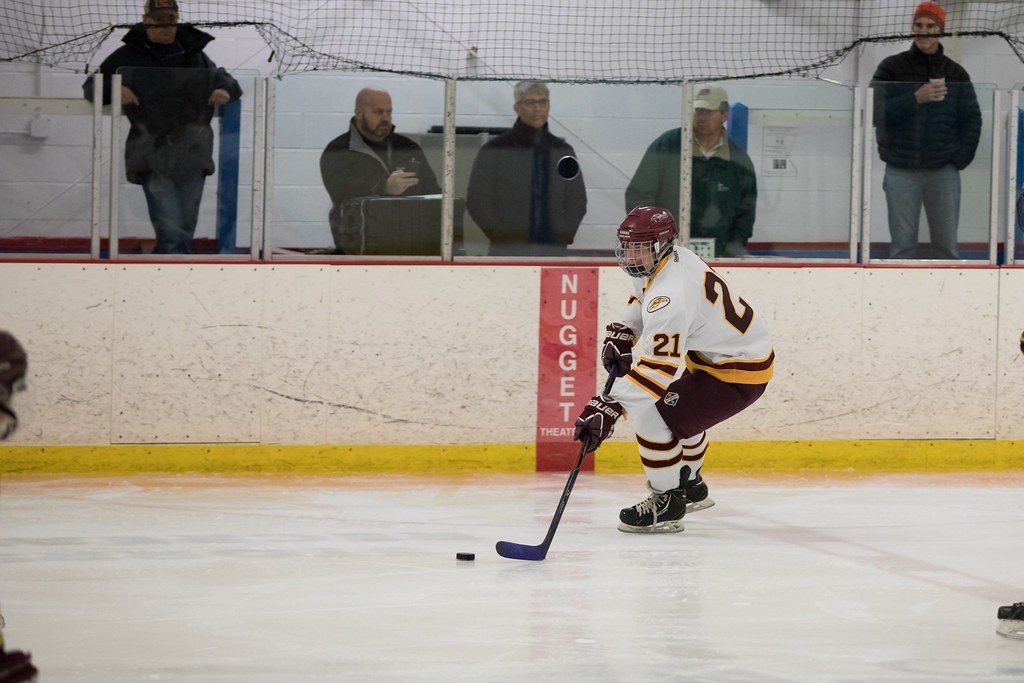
692, 83, 728, 110
144, 0, 179, 21
914, 2, 945, 33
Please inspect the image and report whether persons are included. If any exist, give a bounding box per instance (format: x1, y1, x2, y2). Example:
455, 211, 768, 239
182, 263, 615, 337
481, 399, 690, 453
997, 331, 1024, 635
0, 331, 39, 683
869, 3, 982, 261
320, 86, 444, 252
83, 0, 243, 254
465, 80, 588, 256
625, 82, 756, 258
574, 205, 774, 534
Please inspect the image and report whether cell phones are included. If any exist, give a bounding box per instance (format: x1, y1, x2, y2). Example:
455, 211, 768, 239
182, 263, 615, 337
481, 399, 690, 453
402, 159, 423, 178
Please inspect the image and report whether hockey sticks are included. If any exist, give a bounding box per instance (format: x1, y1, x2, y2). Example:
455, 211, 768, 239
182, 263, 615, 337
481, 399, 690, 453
494, 361, 618, 561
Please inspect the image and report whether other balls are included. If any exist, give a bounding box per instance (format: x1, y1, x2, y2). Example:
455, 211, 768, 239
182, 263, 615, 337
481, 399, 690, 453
456, 552, 475, 561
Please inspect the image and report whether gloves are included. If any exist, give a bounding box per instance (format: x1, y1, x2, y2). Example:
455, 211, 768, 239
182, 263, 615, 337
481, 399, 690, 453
601, 322, 635, 378
573, 396, 623, 454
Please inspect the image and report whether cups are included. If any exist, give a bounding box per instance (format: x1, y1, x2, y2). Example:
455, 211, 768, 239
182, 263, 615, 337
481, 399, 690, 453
929, 78, 945, 98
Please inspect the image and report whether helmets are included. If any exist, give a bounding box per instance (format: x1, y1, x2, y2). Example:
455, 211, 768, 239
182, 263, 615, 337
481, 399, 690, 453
0, 329, 28, 403
615, 205, 679, 277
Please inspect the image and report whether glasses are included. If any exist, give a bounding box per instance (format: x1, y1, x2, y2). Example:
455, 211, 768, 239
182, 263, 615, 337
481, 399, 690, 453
517, 98, 550, 106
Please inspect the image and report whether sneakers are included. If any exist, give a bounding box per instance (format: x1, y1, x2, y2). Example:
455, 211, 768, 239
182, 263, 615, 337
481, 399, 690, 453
685, 466, 715, 514
617, 464, 692, 534
995, 602, 1024, 640
